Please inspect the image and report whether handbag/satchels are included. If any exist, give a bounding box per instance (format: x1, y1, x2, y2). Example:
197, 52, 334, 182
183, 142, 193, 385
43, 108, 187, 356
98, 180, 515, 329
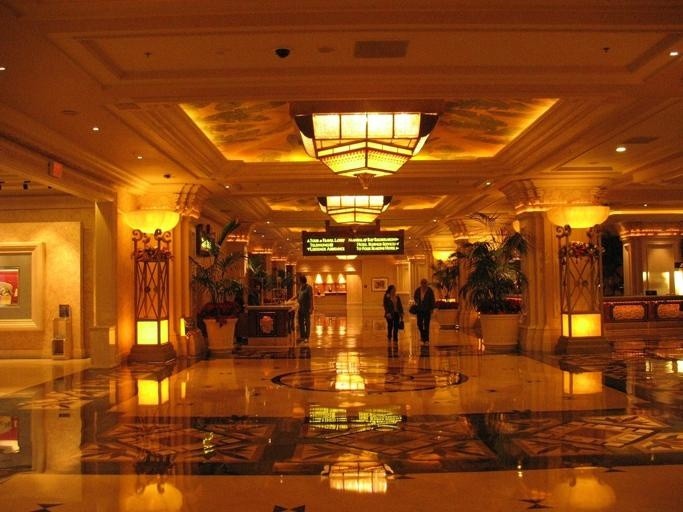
409, 304, 417, 314
399, 321, 404, 329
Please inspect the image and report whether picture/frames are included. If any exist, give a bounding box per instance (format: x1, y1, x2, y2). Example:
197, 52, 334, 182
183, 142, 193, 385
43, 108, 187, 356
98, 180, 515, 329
370, 277, 389, 293
0, 242, 47, 332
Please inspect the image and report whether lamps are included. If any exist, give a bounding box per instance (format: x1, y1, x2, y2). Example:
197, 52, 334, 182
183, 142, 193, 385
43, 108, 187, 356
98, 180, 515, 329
291, 102, 441, 234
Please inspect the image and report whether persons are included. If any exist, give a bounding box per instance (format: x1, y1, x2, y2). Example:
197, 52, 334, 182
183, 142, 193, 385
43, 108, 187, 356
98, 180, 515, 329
414, 278, 435, 347
384, 285, 404, 342
247, 283, 261, 306
289, 276, 313, 343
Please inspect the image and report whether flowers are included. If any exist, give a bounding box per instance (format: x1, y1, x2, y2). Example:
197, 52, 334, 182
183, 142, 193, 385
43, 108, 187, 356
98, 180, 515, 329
557, 241, 606, 268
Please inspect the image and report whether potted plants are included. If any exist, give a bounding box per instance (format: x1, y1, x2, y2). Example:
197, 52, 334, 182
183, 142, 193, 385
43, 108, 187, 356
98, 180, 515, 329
190, 218, 254, 355
432, 211, 531, 356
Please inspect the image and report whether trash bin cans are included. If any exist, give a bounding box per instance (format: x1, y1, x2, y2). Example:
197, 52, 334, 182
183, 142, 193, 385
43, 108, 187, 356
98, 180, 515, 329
88, 325, 117, 366
